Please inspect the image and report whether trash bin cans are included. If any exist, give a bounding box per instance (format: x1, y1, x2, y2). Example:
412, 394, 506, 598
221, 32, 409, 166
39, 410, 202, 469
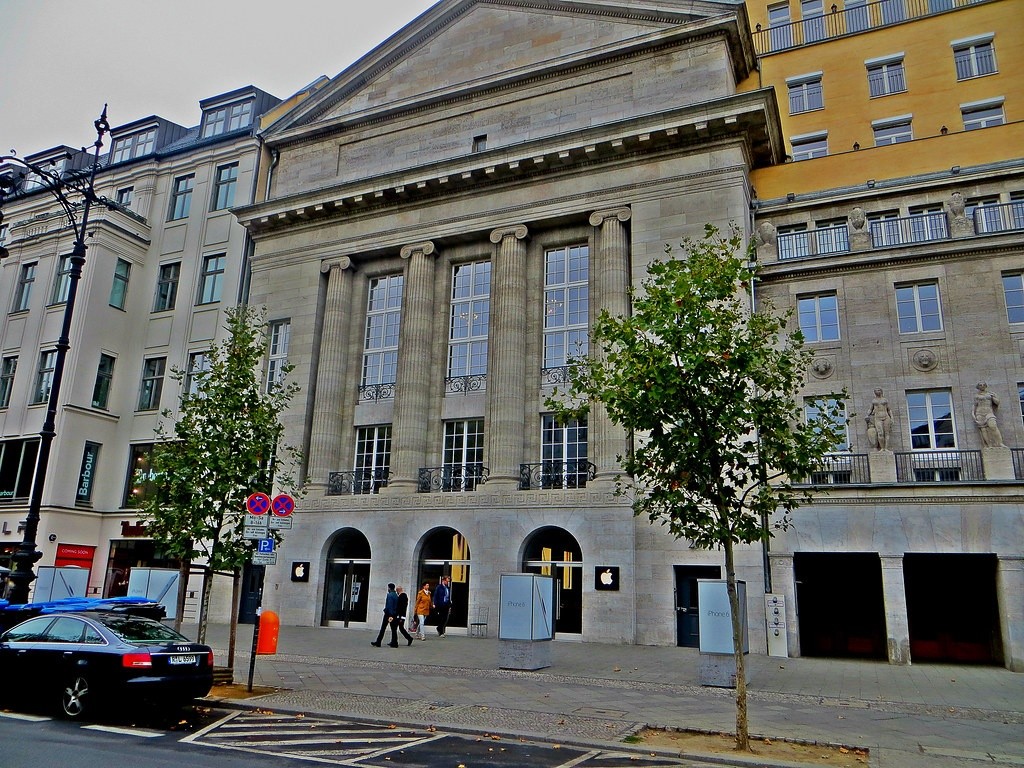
256, 611, 279, 655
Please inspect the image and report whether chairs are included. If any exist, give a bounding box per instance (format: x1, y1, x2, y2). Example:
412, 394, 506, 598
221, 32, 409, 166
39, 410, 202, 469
471, 607, 489, 639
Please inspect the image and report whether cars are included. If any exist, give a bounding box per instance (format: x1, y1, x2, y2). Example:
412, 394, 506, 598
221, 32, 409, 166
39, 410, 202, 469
0, 611, 214, 721
0, 595, 166, 634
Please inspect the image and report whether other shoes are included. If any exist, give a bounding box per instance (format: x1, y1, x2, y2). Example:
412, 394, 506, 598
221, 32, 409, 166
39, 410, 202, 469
371, 642, 381, 647
415, 634, 426, 641
439, 633, 445, 638
407, 637, 414, 646
387, 642, 398, 648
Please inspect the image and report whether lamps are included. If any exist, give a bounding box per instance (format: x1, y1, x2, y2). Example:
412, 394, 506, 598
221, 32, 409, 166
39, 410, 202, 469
950, 165, 960, 175
853, 142, 860, 150
787, 192, 795, 201
867, 179, 876, 188
755, 23, 762, 32
941, 126, 948, 134
831, 4, 837, 13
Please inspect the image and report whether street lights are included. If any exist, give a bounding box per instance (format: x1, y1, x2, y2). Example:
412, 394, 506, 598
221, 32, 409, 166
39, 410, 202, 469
0, 102, 121, 603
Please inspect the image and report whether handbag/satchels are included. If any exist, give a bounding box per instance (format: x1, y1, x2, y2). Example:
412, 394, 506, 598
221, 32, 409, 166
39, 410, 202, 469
408, 614, 419, 632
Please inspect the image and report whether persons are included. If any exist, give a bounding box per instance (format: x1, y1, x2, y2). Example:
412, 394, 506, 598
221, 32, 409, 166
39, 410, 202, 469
867, 387, 894, 452
371, 575, 453, 648
971, 379, 1008, 447
